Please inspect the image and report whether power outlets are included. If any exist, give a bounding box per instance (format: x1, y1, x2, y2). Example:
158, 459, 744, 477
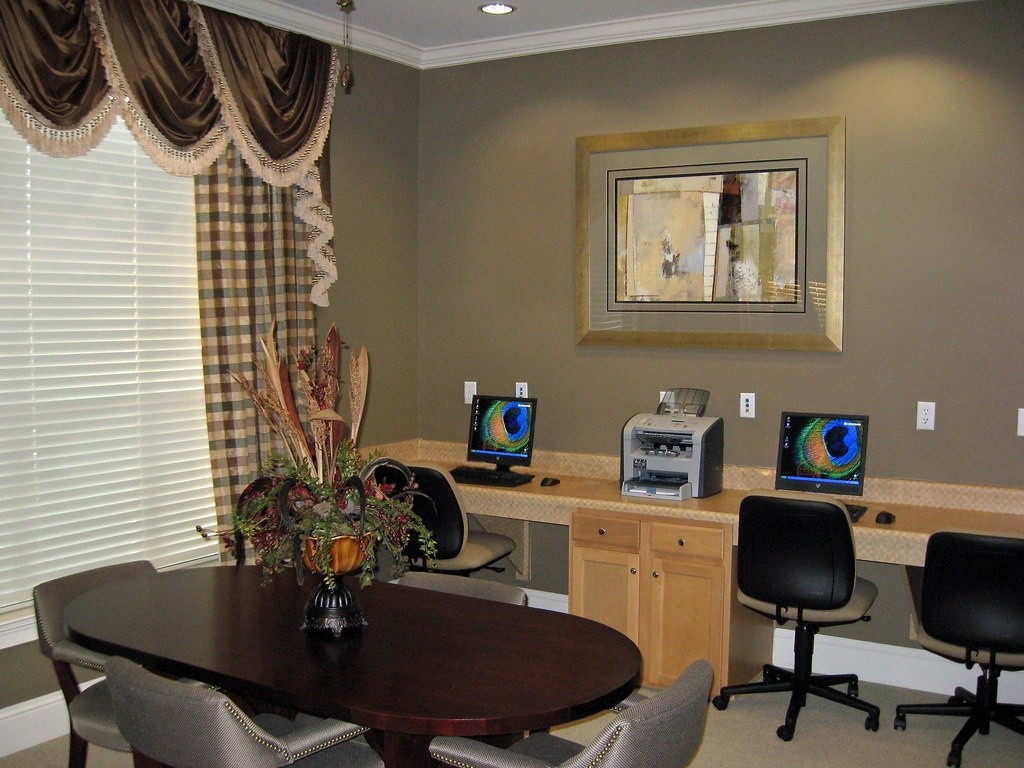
463, 381, 477, 405
915, 401, 936, 431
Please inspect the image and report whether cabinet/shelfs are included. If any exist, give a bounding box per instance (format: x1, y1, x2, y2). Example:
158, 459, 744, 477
567, 507, 774, 701
410, 458, 1024, 569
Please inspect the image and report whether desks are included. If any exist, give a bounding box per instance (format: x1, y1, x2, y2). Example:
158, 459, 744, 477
62, 564, 643, 768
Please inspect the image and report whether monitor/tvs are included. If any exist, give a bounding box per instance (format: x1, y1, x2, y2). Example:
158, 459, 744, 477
775, 411, 869, 496
468, 395, 537, 471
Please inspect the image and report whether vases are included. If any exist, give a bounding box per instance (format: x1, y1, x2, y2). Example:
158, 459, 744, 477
297, 531, 369, 638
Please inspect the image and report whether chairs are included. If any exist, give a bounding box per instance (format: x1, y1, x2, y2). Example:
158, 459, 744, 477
712, 489, 881, 741
429, 658, 715, 768
34, 559, 156, 768
104, 656, 386, 768
373, 458, 516, 578
893, 530, 1024, 768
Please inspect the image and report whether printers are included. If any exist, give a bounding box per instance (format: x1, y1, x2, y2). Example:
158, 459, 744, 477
619, 388, 724, 499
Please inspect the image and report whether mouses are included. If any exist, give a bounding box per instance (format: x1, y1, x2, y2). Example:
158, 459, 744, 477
541, 477, 560, 487
876, 511, 896, 524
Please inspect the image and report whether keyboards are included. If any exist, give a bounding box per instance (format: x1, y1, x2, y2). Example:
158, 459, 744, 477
450, 466, 535, 488
844, 504, 868, 523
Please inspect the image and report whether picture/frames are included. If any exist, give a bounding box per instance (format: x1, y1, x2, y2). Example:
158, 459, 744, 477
574, 116, 845, 352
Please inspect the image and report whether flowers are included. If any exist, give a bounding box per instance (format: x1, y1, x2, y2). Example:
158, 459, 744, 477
193, 317, 436, 590
